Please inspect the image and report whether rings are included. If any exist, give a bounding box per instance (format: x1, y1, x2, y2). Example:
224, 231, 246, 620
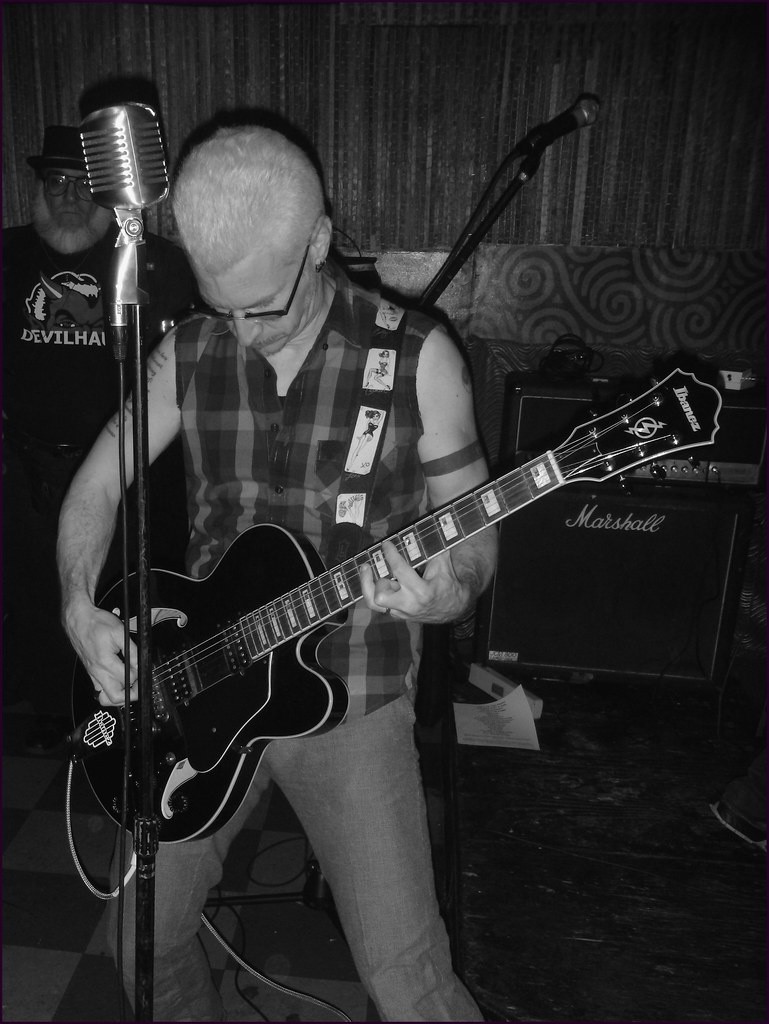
92, 688, 104, 700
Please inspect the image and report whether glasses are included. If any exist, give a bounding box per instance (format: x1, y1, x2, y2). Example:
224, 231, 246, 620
44, 170, 93, 200
191, 236, 311, 322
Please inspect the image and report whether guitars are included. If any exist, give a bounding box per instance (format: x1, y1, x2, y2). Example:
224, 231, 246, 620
69, 367, 723, 845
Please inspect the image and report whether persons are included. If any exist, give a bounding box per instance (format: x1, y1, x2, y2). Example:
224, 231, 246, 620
0, 119, 210, 753
56, 125, 500, 1024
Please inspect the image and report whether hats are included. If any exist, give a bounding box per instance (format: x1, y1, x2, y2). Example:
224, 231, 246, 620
26, 125, 89, 177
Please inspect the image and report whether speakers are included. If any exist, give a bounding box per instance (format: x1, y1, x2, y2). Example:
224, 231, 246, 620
472, 462, 756, 697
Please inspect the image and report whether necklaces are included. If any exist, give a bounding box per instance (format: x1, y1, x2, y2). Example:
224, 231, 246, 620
40, 239, 96, 281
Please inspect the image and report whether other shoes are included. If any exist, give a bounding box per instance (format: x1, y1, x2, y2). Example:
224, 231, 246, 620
711, 798, 769, 850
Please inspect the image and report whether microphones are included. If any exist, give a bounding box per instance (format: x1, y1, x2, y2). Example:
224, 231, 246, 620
513, 98, 600, 158
78, 102, 170, 362
